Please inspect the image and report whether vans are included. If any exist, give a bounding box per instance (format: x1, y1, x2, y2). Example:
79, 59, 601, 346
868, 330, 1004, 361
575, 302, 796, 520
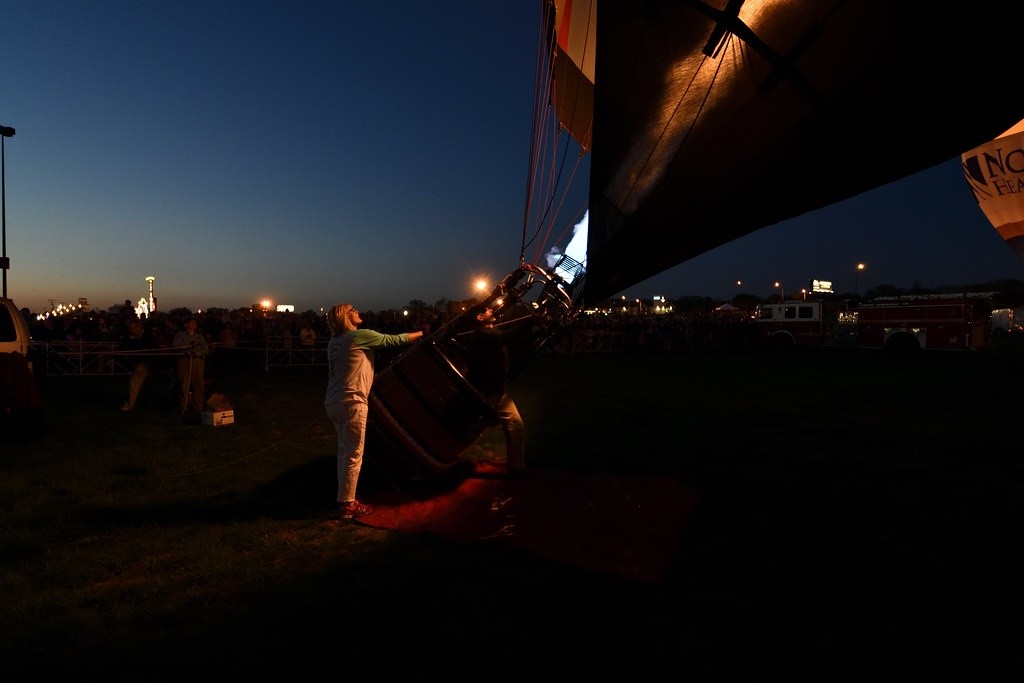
748, 302, 826, 347
0, 295, 43, 436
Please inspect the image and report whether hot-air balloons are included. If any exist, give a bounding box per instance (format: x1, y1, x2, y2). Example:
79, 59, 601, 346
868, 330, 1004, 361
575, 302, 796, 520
363, 0, 1024, 481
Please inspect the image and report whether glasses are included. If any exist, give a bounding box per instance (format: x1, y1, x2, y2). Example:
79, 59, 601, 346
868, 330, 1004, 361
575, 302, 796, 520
346, 307, 356, 315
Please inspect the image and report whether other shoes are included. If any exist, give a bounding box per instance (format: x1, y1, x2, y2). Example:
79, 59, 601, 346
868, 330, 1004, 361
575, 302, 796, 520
340, 500, 376, 518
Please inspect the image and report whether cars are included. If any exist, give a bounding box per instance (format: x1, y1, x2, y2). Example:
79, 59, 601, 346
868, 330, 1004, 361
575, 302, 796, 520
832, 323, 858, 333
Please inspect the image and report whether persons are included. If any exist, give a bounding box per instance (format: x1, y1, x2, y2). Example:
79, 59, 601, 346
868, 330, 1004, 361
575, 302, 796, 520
325, 304, 430, 521
466, 310, 538, 480
22, 300, 763, 424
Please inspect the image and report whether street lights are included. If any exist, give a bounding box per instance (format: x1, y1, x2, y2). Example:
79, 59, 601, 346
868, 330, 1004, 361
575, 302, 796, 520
0, 124, 17, 300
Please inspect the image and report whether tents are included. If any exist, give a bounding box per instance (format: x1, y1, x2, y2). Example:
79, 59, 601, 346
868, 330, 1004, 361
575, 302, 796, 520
715, 303, 734, 312
